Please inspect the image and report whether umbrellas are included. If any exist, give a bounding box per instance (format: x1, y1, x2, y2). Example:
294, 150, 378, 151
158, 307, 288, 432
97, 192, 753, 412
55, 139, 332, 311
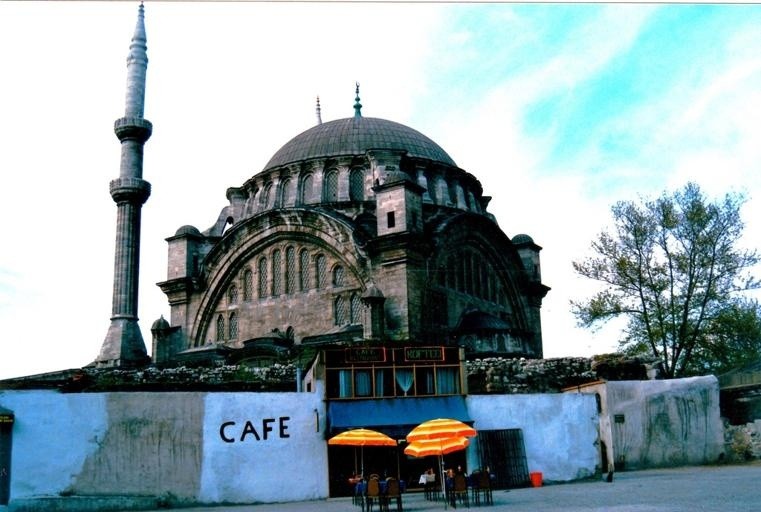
404, 437, 469, 490
327, 428, 397, 479
405, 417, 478, 510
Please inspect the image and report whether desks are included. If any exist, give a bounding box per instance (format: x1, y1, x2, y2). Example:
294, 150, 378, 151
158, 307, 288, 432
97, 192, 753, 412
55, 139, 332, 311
355, 479, 404, 512
420, 474, 496, 508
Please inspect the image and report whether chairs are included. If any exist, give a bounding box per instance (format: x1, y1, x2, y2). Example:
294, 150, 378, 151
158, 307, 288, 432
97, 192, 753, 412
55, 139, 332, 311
450, 469, 492, 508
365, 472, 402, 511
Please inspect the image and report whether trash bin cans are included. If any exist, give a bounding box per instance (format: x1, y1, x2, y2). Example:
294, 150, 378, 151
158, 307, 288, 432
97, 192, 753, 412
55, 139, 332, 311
531, 472, 543, 488
